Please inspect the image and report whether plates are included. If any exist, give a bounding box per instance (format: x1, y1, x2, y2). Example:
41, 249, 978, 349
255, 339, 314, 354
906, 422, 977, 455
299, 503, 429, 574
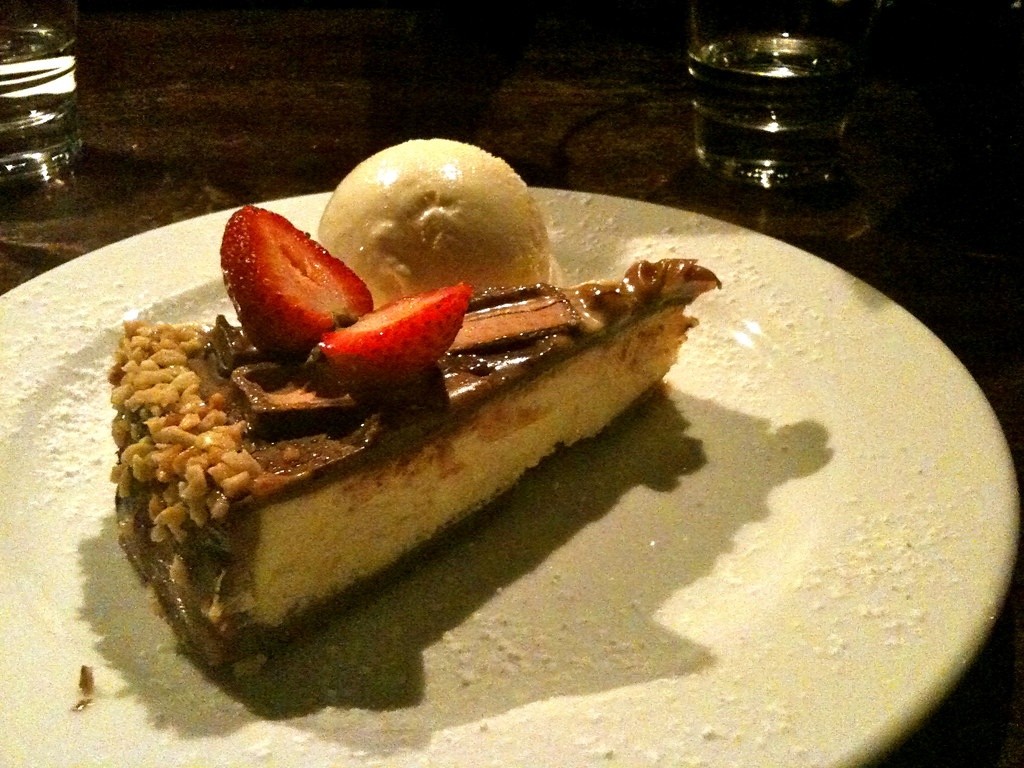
1, 186, 1020, 767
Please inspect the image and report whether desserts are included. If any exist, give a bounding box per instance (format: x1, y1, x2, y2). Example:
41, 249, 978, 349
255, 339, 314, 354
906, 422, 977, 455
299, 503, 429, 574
109, 207, 722, 672
316, 138, 551, 308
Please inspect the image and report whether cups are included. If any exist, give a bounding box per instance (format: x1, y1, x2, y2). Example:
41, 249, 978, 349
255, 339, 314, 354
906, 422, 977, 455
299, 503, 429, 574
688, 0, 894, 193
0, 0, 89, 204
